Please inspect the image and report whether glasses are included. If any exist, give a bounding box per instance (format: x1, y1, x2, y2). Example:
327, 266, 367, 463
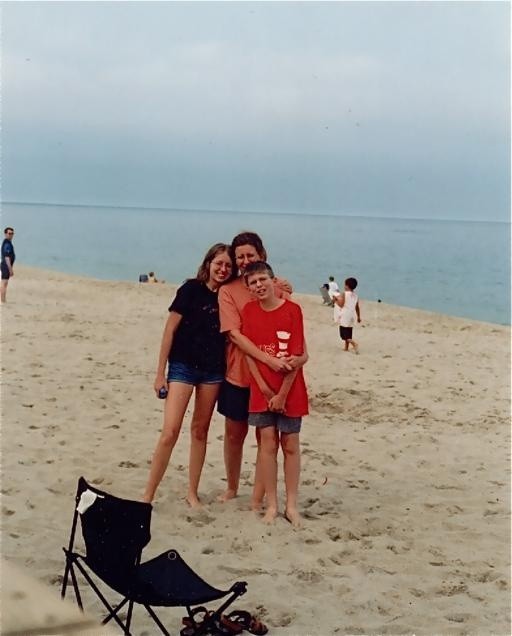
6, 232, 13, 235
209, 260, 233, 269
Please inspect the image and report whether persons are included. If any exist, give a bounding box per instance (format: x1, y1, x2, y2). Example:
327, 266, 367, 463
327, 275, 341, 301
215, 230, 312, 515
0, 226, 17, 305
147, 272, 166, 283
134, 242, 294, 512
237, 260, 311, 527
331, 277, 362, 354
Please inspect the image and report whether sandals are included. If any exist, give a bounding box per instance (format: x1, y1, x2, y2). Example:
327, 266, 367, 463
178, 605, 269, 636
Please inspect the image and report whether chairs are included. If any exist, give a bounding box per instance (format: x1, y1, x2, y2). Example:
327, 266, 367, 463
59, 476, 249, 636
